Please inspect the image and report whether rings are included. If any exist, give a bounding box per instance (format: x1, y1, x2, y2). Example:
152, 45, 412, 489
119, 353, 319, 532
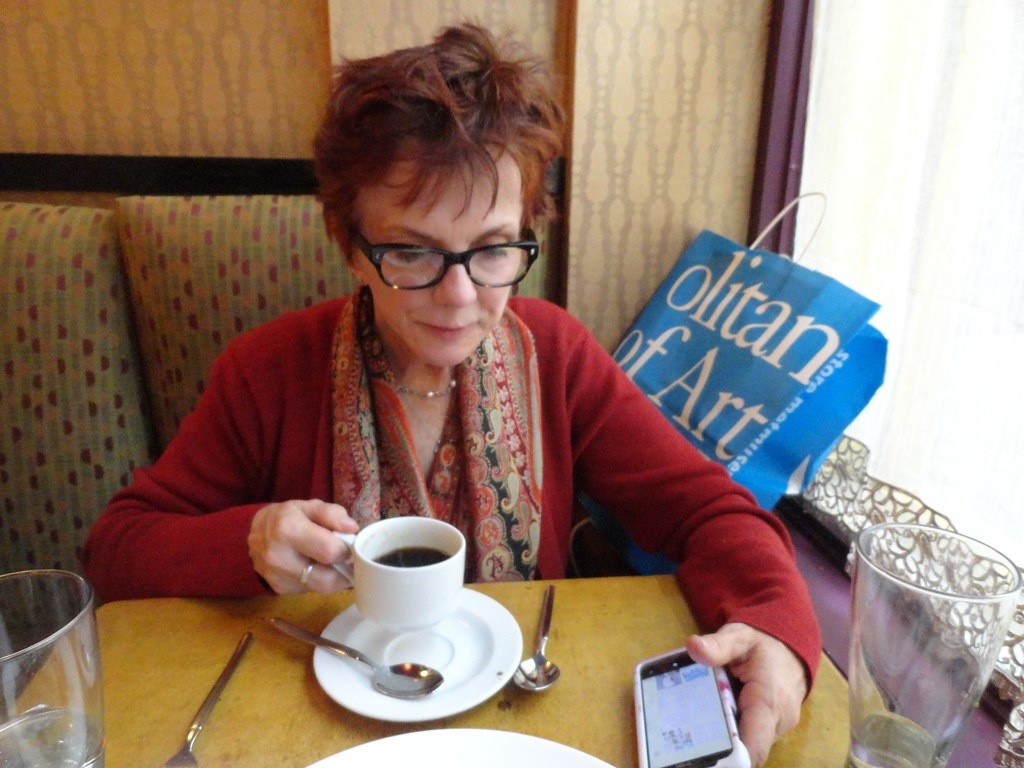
300, 563, 315, 581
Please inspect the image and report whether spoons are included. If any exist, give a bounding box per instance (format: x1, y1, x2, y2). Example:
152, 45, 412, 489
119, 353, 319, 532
271, 616, 444, 699
513, 584, 560, 689
158, 631, 251, 768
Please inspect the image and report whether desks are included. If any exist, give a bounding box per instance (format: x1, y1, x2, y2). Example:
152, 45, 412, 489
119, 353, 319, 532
0, 570, 902, 768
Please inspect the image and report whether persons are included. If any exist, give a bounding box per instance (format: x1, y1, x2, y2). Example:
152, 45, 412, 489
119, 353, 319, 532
81, 24, 822, 767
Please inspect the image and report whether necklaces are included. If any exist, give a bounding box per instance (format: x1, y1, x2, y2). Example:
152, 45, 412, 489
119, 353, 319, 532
396, 381, 458, 399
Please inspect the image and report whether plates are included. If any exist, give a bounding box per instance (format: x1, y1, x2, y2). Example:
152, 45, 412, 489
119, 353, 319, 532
313, 587, 523, 723
306, 728, 615, 768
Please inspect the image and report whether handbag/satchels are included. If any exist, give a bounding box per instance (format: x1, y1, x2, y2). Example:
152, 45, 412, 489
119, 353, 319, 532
570, 191, 888, 573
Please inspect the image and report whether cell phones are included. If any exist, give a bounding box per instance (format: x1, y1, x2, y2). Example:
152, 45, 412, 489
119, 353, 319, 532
634, 646, 750, 768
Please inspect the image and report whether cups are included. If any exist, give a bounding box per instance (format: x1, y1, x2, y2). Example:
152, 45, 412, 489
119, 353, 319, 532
332, 516, 466, 630
846, 522, 1022, 768
0, 569, 107, 768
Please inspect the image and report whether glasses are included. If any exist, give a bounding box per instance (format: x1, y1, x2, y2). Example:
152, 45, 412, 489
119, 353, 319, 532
347, 225, 539, 290
